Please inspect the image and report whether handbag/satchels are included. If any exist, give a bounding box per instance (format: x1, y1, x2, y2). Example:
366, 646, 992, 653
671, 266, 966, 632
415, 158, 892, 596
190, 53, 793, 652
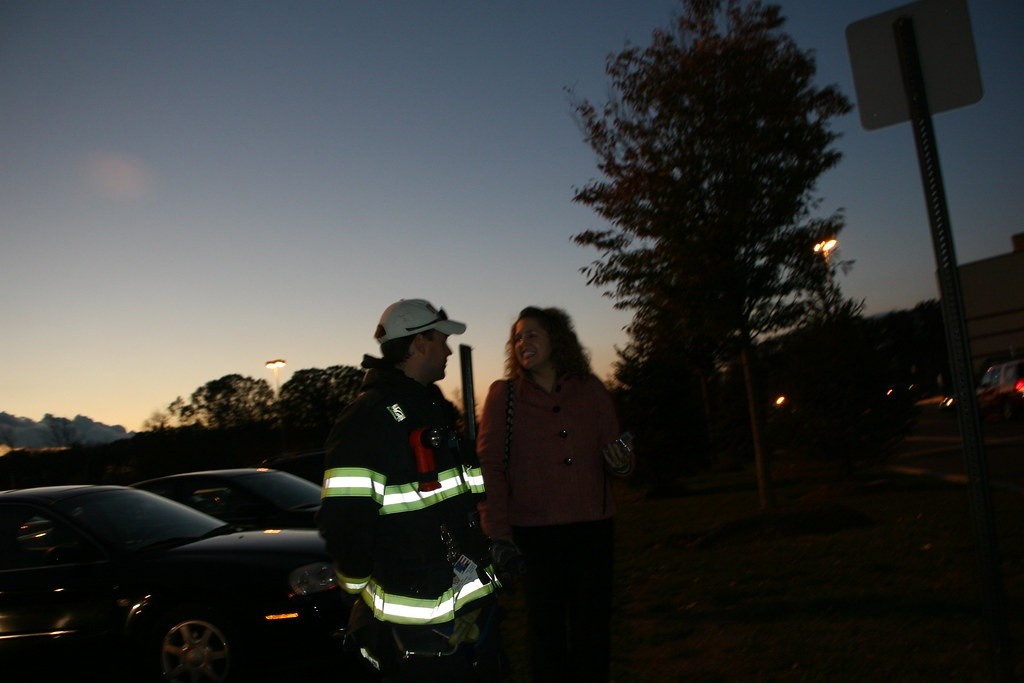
455, 513, 494, 557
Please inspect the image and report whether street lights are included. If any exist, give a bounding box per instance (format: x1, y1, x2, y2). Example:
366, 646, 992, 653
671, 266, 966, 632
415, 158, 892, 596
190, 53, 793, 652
264, 358, 287, 400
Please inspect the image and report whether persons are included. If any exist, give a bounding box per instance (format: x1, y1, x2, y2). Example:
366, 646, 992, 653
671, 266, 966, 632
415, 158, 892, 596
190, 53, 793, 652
322, 301, 524, 683
477, 307, 637, 683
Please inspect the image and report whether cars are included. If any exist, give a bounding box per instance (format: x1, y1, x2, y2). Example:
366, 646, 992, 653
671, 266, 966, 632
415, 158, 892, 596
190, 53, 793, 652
260, 448, 327, 487
16, 465, 324, 561
978, 358, 1024, 425
1, 484, 350, 683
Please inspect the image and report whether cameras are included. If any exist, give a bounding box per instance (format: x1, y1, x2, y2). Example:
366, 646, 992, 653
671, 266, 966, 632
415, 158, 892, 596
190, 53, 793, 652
605, 430, 635, 455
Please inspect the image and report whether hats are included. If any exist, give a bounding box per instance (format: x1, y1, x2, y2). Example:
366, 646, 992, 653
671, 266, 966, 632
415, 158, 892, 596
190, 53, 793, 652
375, 299, 466, 344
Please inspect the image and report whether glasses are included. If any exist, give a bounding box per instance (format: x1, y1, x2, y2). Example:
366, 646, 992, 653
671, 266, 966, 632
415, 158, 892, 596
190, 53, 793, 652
404, 309, 447, 331
